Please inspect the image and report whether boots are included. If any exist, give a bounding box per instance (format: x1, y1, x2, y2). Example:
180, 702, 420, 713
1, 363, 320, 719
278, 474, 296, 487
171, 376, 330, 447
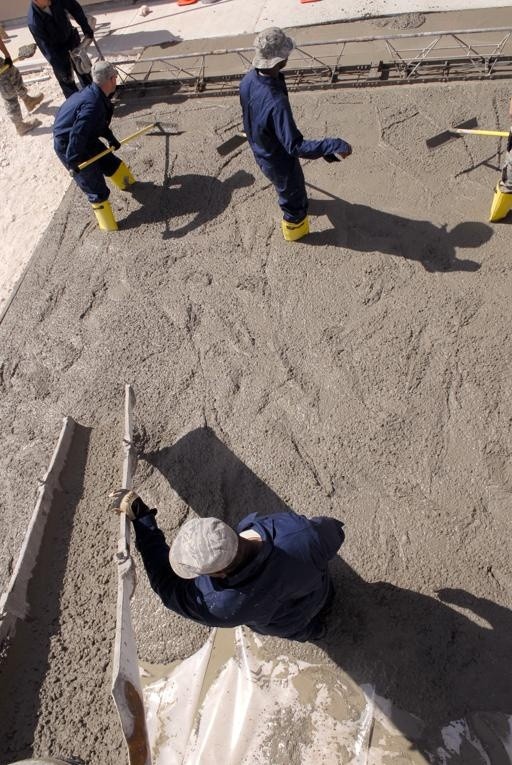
23, 92, 43, 111
13, 118, 38, 135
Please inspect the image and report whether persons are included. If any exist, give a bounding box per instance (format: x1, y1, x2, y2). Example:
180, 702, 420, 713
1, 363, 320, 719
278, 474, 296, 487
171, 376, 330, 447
0, 36, 48, 139
104, 483, 345, 647
50, 60, 137, 235
24, 0, 96, 98
237, 25, 353, 242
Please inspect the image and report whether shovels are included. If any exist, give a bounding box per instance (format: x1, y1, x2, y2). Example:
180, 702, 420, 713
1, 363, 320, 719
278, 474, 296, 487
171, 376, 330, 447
0, 43, 36, 74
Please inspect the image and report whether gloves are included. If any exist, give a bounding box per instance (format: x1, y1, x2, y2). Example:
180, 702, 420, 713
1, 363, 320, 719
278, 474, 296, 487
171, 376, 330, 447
108, 136, 121, 150
82, 24, 93, 39
67, 162, 82, 176
61, 72, 72, 83
109, 489, 151, 520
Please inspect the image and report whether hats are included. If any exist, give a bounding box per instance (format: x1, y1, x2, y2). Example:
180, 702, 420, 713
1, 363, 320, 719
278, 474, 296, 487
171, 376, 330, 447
252, 27, 293, 69
169, 517, 239, 580
91, 61, 118, 81
